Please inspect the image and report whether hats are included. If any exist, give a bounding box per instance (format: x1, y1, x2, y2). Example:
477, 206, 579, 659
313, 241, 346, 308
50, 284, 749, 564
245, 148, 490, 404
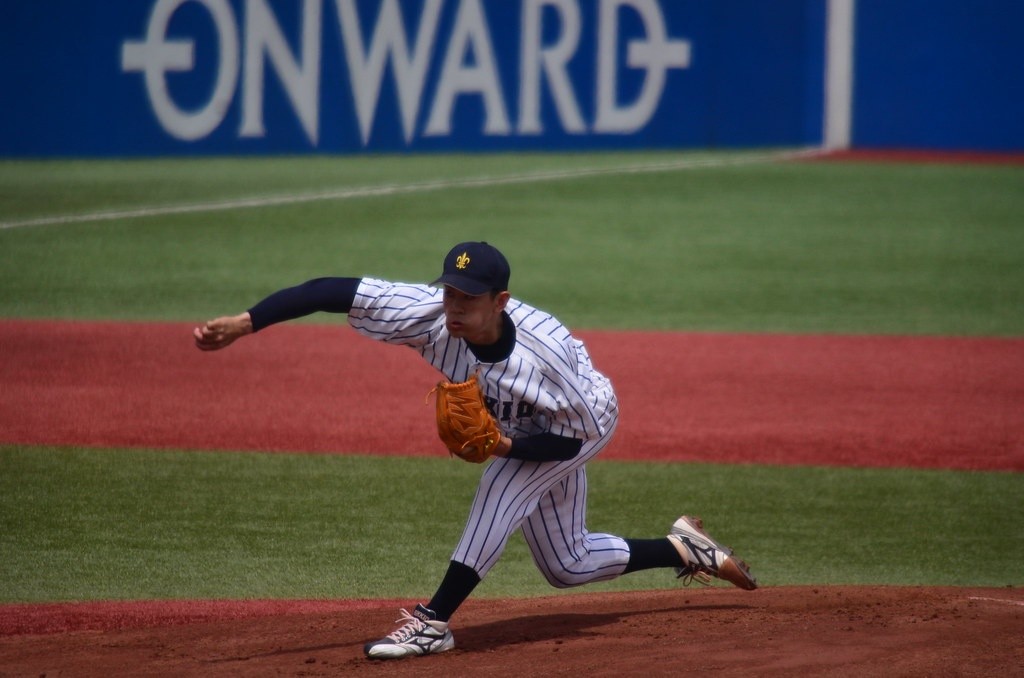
430, 241, 510, 296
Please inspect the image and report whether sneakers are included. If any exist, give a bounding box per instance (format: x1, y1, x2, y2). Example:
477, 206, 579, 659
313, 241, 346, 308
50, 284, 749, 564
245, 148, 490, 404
363, 603, 455, 659
670, 515, 760, 590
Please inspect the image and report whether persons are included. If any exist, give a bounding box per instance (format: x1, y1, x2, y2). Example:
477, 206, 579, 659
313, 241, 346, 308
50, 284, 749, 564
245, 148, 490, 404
192, 243, 758, 659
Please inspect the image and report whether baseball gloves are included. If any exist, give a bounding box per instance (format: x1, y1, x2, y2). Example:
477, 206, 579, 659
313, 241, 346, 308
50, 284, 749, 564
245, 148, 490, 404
435, 374, 502, 465
